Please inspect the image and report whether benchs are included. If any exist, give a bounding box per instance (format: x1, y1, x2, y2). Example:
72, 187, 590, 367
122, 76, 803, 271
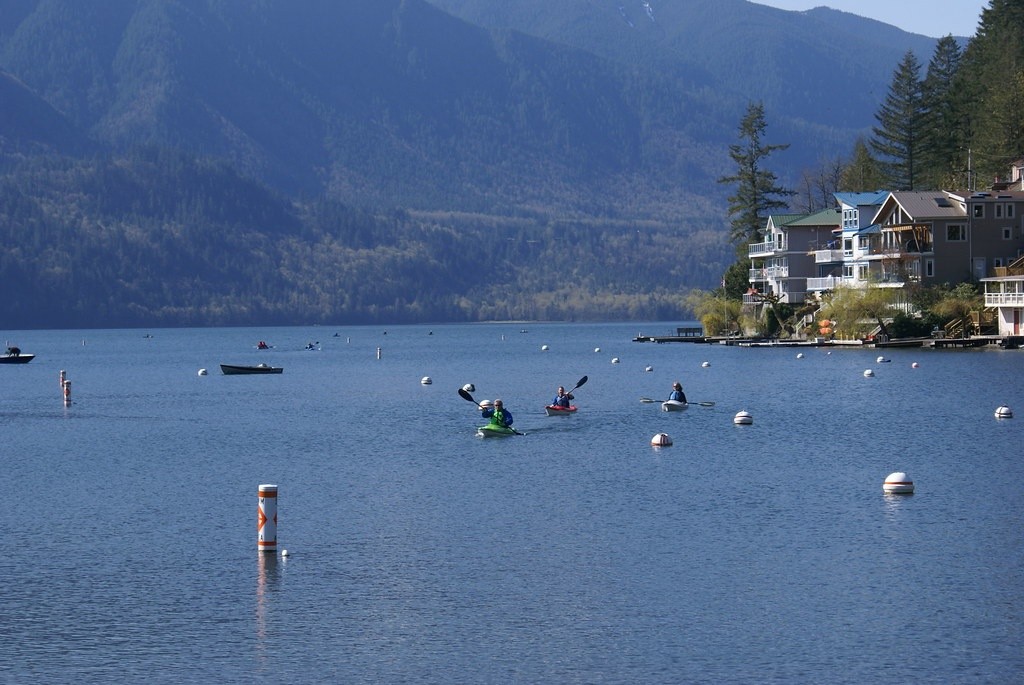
677, 328, 702, 337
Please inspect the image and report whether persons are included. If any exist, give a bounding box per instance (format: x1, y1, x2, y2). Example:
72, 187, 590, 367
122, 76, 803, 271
7, 346, 21, 356
482, 399, 513, 428
662, 382, 687, 403
550, 387, 574, 407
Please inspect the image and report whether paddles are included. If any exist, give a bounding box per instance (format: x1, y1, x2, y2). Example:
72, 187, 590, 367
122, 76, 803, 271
544, 375, 589, 414
639, 396, 716, 408
252, 345, 277, 349
305, 341, 320, 349
457, 388, 524, 435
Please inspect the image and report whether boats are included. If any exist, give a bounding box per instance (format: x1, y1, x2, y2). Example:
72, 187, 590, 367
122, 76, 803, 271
478, 424, 516, 436
257, 345, 268, 350
662, 401, 688, 412
0, 354, 35, 364
333, 334, 340, 338
545, 404, 577, 416
305, 347, 314, 351
220, 363, 283, 375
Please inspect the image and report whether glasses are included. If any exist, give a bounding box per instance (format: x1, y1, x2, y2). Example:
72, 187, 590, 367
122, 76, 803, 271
495, 404, 501, 406
